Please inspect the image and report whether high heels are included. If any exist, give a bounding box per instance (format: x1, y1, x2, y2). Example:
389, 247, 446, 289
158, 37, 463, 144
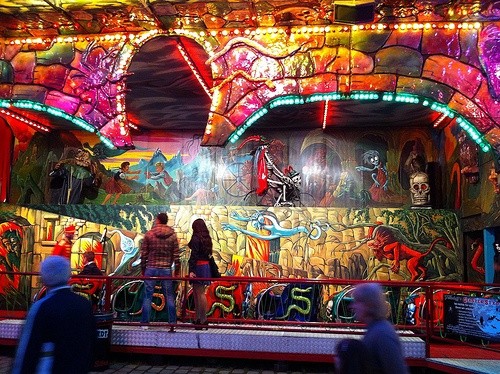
195, 320, 208, 330
192, 318, 201, 324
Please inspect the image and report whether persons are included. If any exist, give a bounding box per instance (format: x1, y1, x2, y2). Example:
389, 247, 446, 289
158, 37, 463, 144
11, 255, 97, 374
352, 283, 408, 374
77, 251, 103, 276
335, 339, 375, 374
140, 213, 180, 330
187, 219, 213, 330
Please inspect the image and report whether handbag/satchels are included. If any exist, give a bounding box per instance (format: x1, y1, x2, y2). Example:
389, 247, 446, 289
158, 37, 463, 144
210, 256, 221, 281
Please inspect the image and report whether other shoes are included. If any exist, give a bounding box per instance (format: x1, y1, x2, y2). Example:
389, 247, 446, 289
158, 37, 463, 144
137, 326, 148, 330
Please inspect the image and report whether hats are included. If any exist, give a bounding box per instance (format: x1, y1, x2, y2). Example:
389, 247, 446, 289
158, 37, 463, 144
64, 226, 75, 234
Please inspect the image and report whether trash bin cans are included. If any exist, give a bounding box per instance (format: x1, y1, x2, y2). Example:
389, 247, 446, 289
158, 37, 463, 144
93, 312, 115, 371
